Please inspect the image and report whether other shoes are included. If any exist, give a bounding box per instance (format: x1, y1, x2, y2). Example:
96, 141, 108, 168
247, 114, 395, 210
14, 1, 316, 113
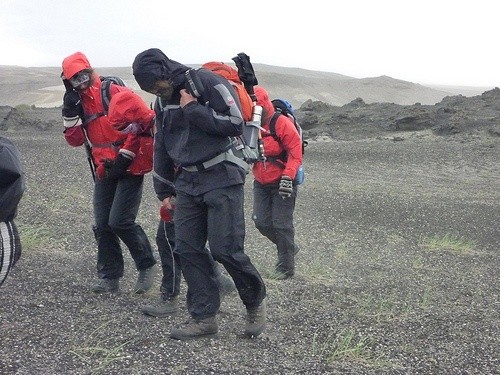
271, 270, 291, 280
292, 244, 300, 256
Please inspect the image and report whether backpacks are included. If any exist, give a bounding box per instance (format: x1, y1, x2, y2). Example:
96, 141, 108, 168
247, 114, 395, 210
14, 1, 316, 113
159, 60, 260, 162
272, 98, 305, 162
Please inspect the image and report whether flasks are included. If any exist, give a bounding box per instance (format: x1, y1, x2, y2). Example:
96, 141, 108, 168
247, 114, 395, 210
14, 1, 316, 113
251, 104, 262, 121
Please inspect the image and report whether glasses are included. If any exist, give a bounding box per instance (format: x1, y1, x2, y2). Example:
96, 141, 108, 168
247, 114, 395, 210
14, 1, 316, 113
70, 71, 90, 87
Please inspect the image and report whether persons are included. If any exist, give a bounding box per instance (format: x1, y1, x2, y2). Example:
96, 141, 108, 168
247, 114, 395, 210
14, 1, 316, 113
109, 48, 266, 340
252, 85, 308, 281
61, 52, 153, 293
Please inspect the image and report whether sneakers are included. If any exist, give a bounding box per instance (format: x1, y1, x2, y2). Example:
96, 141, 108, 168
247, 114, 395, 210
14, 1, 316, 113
91, 278, 119, 293
134, 264, 158, 294
141, 287, 179, 318
245, 295, 268, 337
216, 273, 235, 303
170, 314, 219, 340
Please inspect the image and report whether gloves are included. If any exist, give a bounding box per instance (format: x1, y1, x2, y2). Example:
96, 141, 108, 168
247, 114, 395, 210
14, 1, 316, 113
278, 175, 292, 199
100, 153, 133, 180
62, 89, 82, 117
